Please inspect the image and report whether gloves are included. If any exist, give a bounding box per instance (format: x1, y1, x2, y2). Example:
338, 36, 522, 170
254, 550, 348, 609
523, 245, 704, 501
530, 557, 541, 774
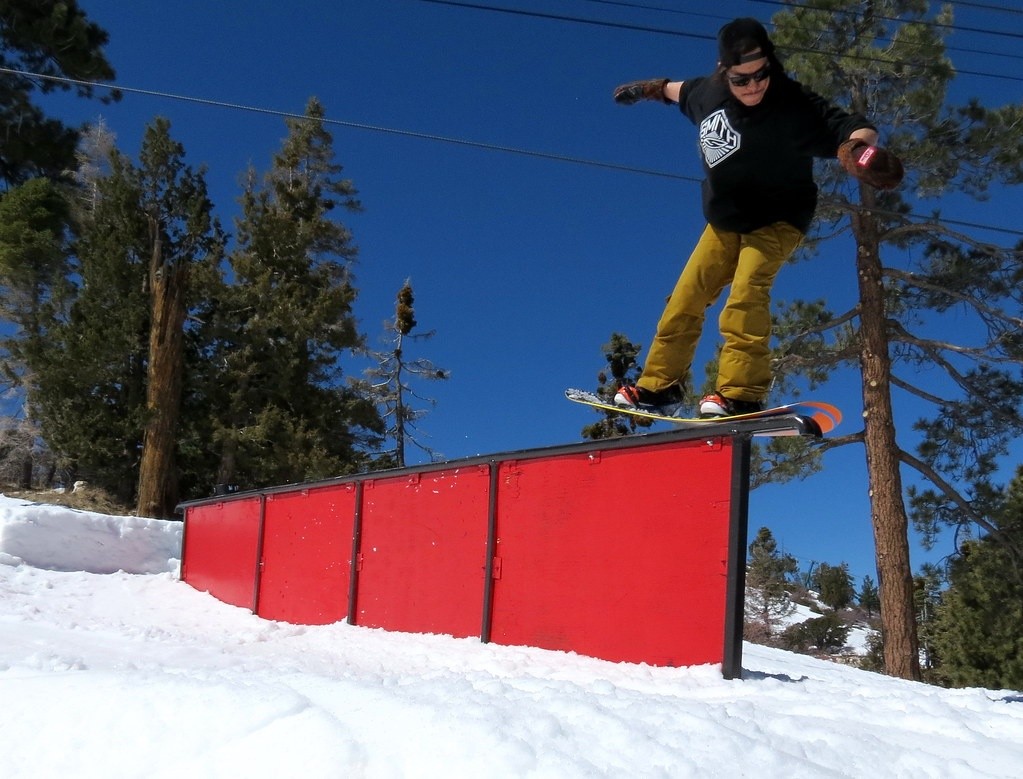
837, 138, 903, 190
613, 78, 673, 106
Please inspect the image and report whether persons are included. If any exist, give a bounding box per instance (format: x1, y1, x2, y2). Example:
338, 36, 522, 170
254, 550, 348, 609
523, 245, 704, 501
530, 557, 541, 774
614, 19, 904, 418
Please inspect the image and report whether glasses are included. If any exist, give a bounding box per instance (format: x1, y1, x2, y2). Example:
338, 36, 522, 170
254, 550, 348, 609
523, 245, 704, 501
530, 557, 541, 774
724, 59, 769, 86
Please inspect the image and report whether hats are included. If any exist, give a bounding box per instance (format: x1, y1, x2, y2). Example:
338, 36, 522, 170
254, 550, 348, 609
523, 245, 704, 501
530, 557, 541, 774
717, 17, 773, 67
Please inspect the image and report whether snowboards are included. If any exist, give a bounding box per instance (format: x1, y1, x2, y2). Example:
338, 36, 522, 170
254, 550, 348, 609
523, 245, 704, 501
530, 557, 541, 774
564, 386, 843, 435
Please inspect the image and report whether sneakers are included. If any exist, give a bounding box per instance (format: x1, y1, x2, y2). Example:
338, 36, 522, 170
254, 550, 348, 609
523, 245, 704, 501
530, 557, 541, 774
698, 393, 763, 416
615, 385, 684, 415
